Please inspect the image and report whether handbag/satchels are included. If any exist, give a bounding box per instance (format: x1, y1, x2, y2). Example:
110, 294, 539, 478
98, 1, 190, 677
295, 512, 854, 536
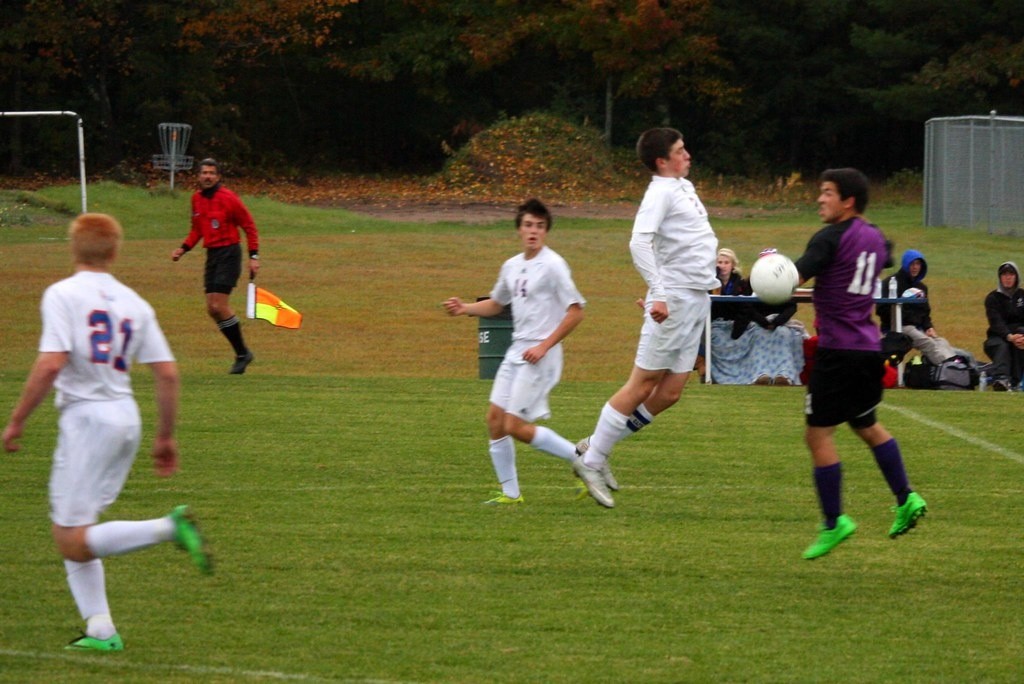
881, 331, 980, 390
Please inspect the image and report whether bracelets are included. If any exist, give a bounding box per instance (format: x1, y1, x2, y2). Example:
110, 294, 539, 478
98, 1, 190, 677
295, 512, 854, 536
251, 255, 258, 259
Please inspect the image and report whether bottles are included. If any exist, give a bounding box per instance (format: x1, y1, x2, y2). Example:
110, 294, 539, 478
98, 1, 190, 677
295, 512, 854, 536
871, 276, 882, 300
979, 372, 986, 392
888, 276, 897, 299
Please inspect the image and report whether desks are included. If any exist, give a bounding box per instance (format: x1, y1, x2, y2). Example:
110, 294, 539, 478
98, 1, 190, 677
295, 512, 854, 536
704, 294, 928, 386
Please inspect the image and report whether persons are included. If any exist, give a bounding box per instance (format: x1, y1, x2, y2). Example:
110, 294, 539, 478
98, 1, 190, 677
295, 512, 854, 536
985, 262, 1024, 392
696, 248, 797, 384
793, 168, 927, 561
2, 213, 213, 653
573, 126, 722, 509
875, 251, 958, 366
442, 198, 588, 504
173, 159, 259, 372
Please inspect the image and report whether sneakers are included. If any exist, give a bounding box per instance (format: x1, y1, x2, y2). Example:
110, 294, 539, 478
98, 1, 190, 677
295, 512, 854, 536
169, 504, 213, 574
484, 491, 524, 505
230, 352, 253, 374
573, 478, 589, 501
888, 493, 929, 540
800, 512, 858, 560
573, 455, 614, 508
65, 630, 124, 653
574, 436, 618, 491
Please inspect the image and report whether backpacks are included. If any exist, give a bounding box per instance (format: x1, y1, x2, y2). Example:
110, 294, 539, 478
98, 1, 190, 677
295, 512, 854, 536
800, 334, 818, 384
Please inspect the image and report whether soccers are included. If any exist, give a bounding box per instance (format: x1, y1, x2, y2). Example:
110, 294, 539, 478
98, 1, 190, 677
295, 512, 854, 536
750, 254, 799, 305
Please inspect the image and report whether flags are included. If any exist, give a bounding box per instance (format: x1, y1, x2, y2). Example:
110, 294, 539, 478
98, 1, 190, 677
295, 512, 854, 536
246, 283, 302, 329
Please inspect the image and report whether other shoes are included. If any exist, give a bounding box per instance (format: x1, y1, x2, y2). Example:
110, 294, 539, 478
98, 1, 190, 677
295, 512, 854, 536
993, 378, 1011, 391
755, 373, 769, 384
774, 374, 788, 385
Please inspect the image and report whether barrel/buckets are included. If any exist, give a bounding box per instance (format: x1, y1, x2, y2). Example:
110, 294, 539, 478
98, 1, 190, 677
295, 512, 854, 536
476, 296, 514, 379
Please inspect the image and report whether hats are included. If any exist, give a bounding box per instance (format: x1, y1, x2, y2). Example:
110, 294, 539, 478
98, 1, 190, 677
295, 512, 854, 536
999, 264, 1016, 274
